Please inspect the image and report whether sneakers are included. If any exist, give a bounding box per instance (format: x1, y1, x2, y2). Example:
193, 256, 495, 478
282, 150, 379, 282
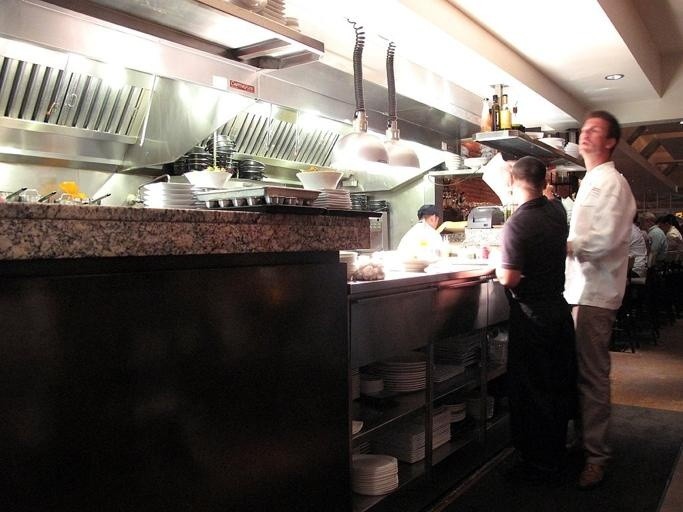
575, 463, 611, 491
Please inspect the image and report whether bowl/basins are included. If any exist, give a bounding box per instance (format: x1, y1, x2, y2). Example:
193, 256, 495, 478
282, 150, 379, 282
463, 155, 488, 170
399, 257, 431, 273
171, 133, 269, 181
295, 170, 344, 190
340, 250, 359, 281
205, 194, 306, 210
182, 169, 234, 189
444, 151, 462, 171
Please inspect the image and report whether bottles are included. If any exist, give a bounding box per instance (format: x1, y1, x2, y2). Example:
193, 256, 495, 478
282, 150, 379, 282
479, 92, 512, 132
505, 189, 515, 220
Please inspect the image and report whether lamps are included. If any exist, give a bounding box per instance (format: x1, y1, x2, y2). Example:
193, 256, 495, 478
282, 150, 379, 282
332, 16, 421, 168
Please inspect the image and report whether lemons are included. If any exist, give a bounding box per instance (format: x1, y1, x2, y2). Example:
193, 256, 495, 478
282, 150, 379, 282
61, 182, 78, 194
72, 193, 85, 198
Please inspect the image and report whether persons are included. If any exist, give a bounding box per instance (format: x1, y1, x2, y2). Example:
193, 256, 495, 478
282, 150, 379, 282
626, 222, 647, 286
486, 155, 573, 495
656, 215, 682, 263
636, 211, 668, 268
397, 204, 444, 259
562, 111, 637, 488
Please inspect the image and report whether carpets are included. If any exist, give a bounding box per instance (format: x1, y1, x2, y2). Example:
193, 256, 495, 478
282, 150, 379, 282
442, 403, 682, 511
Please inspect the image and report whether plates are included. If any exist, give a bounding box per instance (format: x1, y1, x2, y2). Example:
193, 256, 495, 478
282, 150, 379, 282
534, 130, 580, 161
348, 317, 508, 436
350, 385, 495, 497
309, 189, 353, 210
234, 0, 305, 33
353, 195, 388, 211
141, 181, 198, 210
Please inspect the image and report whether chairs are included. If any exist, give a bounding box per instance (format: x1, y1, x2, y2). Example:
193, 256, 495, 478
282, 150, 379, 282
608, 250, 683, 354
